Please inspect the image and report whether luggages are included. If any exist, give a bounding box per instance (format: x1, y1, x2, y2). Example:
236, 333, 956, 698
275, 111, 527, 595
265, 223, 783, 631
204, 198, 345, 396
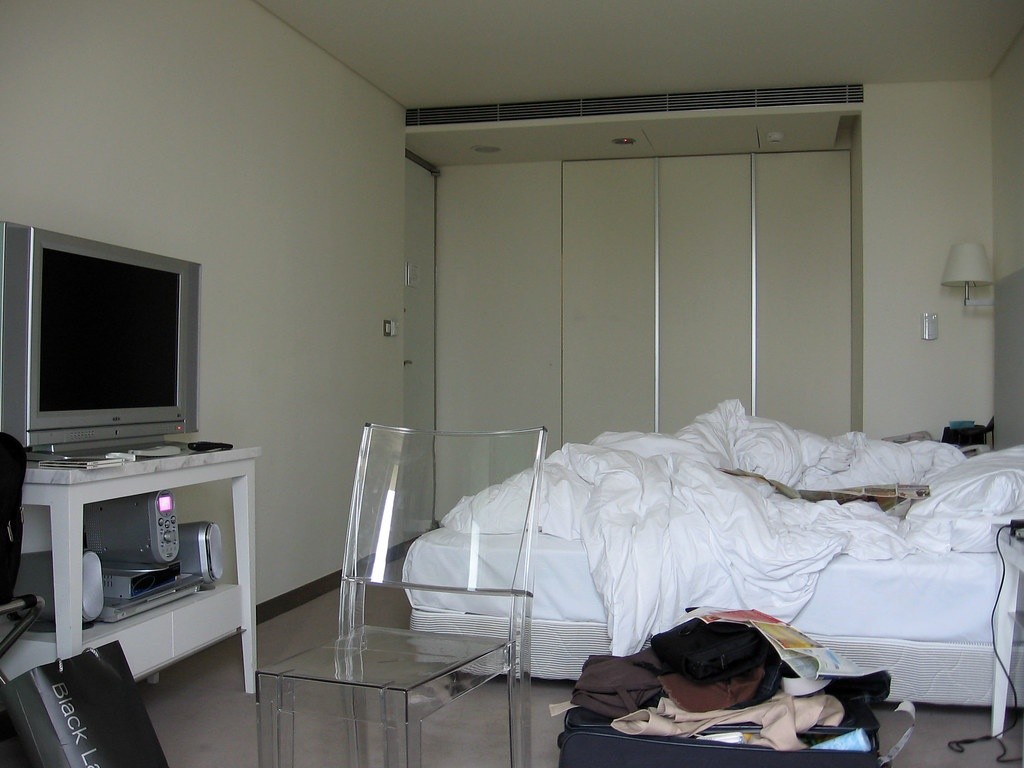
556, 707, 880, 768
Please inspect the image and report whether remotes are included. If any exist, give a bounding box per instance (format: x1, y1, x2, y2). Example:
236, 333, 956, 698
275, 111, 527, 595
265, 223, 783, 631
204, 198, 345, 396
129, 445, 181, 457
188, 440, 234, 452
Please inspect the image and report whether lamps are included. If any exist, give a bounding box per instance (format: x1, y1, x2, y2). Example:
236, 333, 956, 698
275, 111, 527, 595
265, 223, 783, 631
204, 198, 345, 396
941, 242, 1000, 305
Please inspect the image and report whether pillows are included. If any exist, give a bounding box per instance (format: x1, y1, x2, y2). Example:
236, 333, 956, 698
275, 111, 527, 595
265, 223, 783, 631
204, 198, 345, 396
905, 444, 1024, 553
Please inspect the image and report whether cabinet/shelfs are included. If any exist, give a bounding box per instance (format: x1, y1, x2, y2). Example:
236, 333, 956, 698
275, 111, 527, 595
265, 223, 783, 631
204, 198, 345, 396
0, 441, 262, 694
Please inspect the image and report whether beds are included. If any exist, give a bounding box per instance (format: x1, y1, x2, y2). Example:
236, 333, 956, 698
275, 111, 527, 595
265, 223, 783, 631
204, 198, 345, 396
401, 399, 1024, 707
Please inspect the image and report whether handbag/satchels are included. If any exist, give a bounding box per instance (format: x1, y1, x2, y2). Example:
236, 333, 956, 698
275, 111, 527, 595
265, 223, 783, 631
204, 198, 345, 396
651, 618, 769, 684
0, 640, 169, 768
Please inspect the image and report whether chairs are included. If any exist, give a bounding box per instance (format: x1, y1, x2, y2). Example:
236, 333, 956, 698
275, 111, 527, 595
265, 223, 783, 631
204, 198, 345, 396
255, 422, 548, 768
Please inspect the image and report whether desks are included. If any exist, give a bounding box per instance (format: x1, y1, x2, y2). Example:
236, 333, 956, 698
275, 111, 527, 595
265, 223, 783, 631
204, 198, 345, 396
990, 524, 1024, 738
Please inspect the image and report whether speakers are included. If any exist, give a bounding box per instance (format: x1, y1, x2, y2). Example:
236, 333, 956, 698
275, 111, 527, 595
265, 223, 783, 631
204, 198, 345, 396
12, 549, 104, 633
175, 522, 223, 588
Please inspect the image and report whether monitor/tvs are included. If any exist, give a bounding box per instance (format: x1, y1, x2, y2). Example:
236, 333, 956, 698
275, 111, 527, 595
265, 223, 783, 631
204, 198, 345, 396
0, 220, 201, 461
